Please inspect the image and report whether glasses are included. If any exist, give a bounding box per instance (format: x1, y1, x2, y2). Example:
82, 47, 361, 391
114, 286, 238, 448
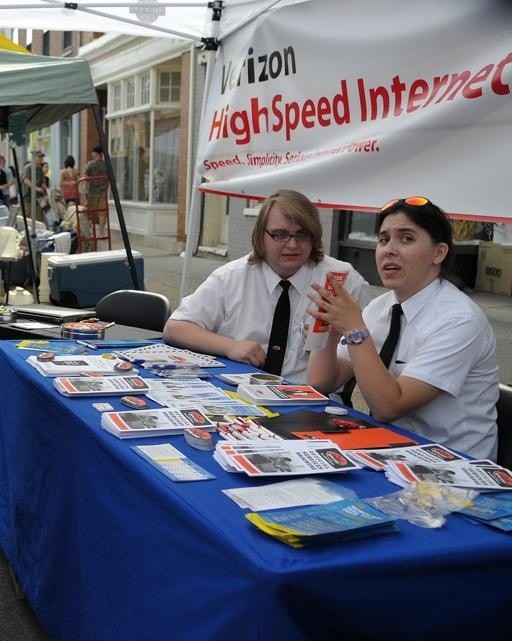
380, 196, 431, 213
263, 228, 314, 242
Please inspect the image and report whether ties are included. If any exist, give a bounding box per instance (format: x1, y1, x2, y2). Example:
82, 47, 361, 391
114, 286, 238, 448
369, 304, 404, 417
262, 280, 291, 376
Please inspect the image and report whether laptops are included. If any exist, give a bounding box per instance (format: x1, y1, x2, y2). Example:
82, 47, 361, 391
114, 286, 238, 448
7, 304, 97, 325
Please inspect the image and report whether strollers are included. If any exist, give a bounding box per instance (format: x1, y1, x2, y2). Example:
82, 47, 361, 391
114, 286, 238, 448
38, 186, 89, 255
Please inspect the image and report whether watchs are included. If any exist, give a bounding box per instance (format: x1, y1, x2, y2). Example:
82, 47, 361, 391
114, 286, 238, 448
341, 328, 371, 345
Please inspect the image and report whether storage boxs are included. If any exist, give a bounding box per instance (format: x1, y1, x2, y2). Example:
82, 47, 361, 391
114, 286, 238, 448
475, 241, 512, 296
45, 249, 146, 306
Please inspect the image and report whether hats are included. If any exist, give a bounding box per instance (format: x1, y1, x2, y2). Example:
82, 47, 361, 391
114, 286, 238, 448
36, 150, 45, 157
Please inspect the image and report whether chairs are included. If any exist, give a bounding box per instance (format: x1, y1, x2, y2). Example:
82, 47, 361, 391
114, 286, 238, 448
95, 290, 170, 332
1, 210, 78, 310
495, 381, 512, 471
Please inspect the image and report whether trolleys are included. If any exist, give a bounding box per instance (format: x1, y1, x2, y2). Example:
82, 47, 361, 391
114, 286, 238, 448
73, 175, 114, 252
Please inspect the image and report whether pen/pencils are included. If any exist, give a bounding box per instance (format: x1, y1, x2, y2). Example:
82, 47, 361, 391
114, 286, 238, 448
76, 339, 97, 350
133, 358, 215, 379
329, 418, 367, 429
104, 322, 115, 329
216, 416, 283, 441
80, 318, 99, 323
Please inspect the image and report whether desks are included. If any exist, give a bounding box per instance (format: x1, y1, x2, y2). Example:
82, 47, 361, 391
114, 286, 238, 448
0, 321, 511, 641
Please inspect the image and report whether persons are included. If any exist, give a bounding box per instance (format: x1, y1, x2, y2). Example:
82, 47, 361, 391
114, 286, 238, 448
84, 145, 109, 239
300, 195, 501, 464
124, 145, 134, 199
126, 146, 149, 200
0, 149, 81, 213
163, 189, 368, 407
